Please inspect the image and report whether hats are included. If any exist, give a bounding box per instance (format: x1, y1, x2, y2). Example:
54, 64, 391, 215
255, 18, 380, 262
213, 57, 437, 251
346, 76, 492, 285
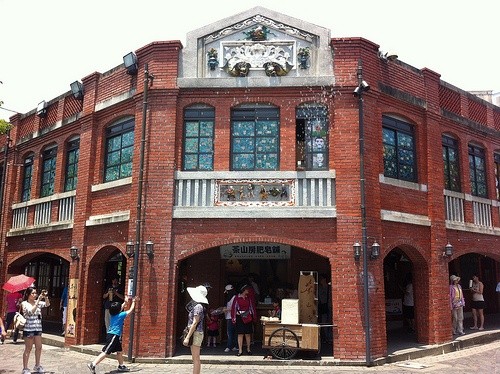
223, 285, 233, 293
450, 275, 461, 283
187, 285, 209, 304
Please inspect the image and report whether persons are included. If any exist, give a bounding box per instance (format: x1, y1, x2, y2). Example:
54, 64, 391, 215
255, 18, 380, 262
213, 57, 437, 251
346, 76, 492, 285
221, 285, 238, 352
87, 296, 140, 374
60, 277, 68, 335
469, 272, 485, 330
268, 297, 281, 319
183, 285, 209, 374
0, 292, 22, 345
205, 311, 219, 348
402, 283, 417, 335
21, 287, 50, 374
450, 275, 466, 335
231, 283, 257, 356
103, 274, 124, 332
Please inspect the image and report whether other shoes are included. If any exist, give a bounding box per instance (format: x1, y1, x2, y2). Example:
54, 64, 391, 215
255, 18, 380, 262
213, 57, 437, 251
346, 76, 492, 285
247, 350, 253, 355
453, 331, 466, 335
469, 326, 485, 331
12, 340, 17, 344
232, 347, 236, 351
206, 344, 211, 347
33, 364, 45, 374
237, 352, 243, 356
224, 347, 230, 352
212, 343, 216, 348
117, 365, 130, 371
22, 368, 32, 374
87, 362, 96, 374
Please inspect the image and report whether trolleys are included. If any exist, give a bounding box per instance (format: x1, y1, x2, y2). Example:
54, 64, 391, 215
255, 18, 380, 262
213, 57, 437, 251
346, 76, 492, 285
259, 318, 338, 360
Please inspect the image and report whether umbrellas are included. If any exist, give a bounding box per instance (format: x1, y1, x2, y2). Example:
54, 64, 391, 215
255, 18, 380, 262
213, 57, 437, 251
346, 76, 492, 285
2, 274, 36, 293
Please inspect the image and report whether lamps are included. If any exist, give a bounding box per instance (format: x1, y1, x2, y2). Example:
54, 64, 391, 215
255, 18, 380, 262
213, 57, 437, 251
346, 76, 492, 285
353, 241, 361, 261
369, 240, 380, 261
37, 100, 47, 119
123, 52, 139, 75
70, 246, 80, 261
126, 240, 134, 258
70, 81, 84, 100
443, 241, 453, 259
146, 238, 154, 260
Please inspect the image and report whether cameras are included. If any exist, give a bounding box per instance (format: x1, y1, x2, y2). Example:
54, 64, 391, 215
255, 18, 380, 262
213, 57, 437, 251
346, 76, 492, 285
42, 290, 46, 296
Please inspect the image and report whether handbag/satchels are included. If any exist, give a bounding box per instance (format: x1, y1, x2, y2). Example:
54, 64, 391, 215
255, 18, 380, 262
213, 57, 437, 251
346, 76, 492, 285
13, 311, 27, 330
180, 327, 193, 346
241, 311, 252, 324
453, 299, 463, 309
